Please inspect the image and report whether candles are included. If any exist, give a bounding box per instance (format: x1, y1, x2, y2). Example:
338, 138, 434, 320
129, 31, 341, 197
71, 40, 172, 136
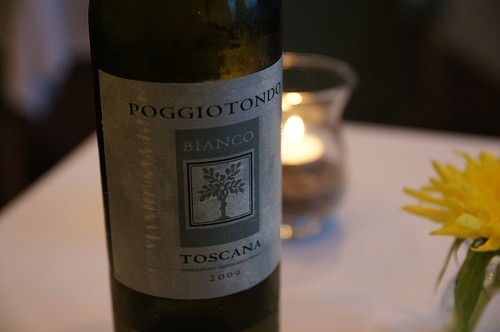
280, 115, 326, 181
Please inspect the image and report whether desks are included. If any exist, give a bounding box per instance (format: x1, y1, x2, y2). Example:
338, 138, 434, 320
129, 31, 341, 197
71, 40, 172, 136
2, 118, 496, 331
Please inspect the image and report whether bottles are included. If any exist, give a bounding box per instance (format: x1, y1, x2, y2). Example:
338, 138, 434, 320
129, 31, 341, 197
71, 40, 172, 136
87, 0, 281, 332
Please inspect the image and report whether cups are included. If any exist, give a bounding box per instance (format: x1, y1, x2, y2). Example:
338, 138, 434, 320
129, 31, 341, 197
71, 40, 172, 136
281, 52, 356, 165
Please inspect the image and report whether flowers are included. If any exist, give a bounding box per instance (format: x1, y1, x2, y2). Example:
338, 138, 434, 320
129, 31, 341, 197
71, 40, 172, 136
397, 148, 497, 332
